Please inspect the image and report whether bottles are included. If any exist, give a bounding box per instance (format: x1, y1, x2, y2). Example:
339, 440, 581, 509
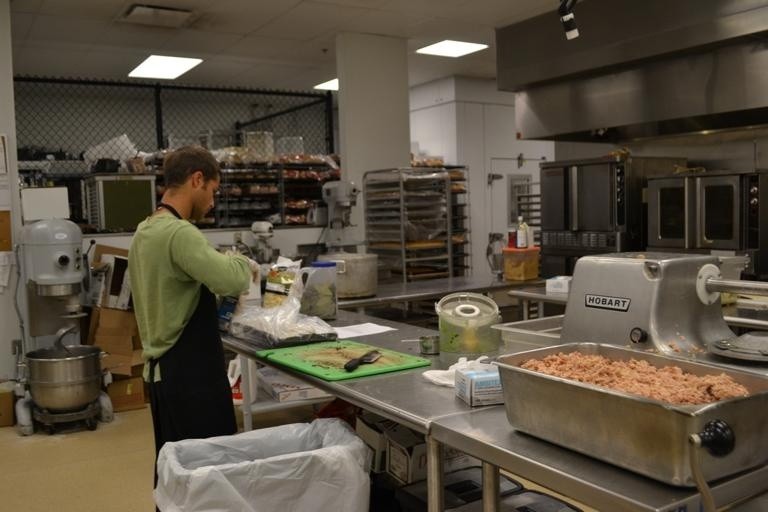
516, 216, 528, 249
312, 199, 328, 227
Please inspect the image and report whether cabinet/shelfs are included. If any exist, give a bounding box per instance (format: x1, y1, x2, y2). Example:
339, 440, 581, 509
414, 164, 471, 317
360, 166, 453, 317
514, 180, 542, 319
85, 147, 342, 230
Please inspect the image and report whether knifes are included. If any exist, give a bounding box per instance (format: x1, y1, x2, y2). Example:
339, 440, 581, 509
343, 348, 381, 371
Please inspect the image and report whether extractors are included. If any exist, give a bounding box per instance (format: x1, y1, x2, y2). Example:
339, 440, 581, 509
493, 0, 768, 147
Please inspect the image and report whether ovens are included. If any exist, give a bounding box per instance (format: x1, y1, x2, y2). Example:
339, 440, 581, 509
642, 170, 768, 254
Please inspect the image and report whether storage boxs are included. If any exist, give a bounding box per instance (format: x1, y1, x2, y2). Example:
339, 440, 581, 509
355, 412, 398, 474
443, 487, 583, 511
86, 243, 152, 414
398, 465, 524, 512
384, 421, 484, 486
0, 378, 17, 426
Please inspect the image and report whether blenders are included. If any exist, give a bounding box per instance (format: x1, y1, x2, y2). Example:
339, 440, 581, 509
12, 220, 115, 436
314, 180, 378, 298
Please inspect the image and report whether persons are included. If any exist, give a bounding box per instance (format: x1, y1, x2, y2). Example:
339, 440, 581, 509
128, 145, 262, 512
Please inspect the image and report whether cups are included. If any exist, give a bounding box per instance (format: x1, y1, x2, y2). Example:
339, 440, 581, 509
420, 336, 439, 355
508, 228, 516, 249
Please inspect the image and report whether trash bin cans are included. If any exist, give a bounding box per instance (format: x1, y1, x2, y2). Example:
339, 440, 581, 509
152, 419, 369, 511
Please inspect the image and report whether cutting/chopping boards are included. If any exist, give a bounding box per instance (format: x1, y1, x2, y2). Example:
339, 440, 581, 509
255, 339, 432, 382
